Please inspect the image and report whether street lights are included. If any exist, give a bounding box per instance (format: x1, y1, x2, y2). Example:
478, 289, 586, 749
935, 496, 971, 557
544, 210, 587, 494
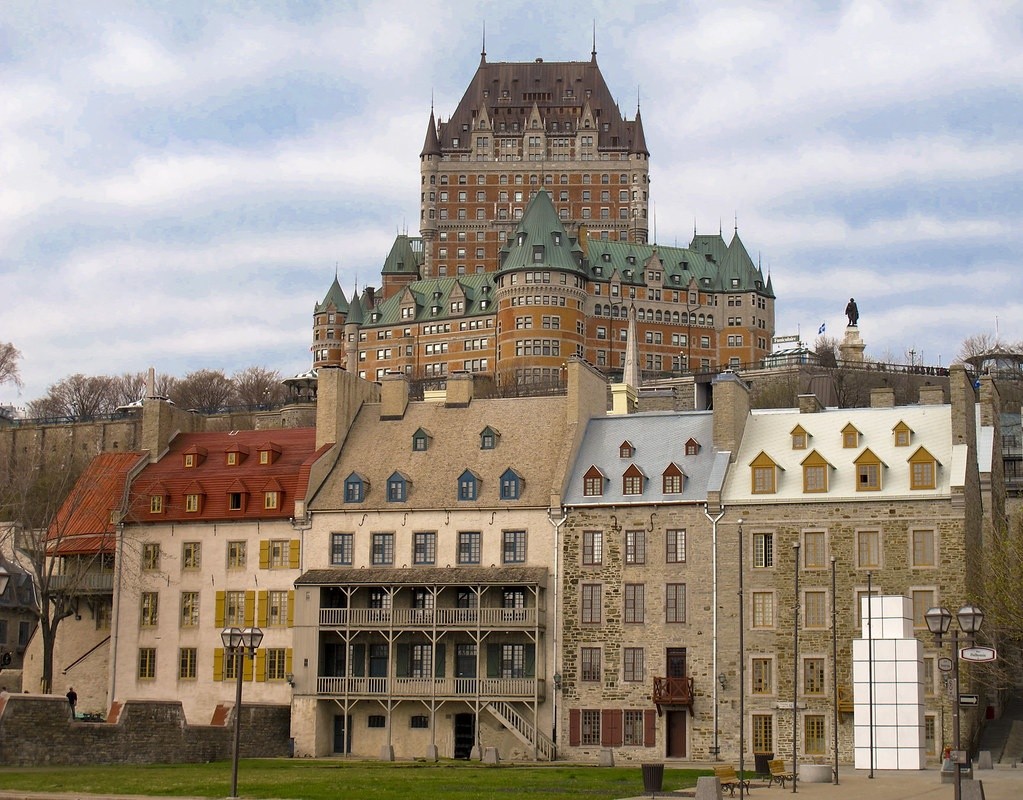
924, 606, 985, 800
221, 627, 264, 798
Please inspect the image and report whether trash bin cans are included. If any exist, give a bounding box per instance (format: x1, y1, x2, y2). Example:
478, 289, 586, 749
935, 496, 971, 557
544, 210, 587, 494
641, 764, 664, 791
754, 752, 774, 775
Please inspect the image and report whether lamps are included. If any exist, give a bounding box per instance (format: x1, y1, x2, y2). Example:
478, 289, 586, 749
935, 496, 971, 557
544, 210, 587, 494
286, 672, 295, 687
718, 672, 727, 691
553, 672, 562, 689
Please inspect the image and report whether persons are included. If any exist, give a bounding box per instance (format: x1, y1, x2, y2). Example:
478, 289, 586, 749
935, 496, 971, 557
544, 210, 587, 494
66, 687, 77, 719
845, 298, 859, 326
1, 687, 9, 695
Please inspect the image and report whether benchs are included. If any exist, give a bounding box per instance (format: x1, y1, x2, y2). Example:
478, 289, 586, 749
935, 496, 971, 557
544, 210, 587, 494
713, 764, 750, 796
767, 760, 800, 789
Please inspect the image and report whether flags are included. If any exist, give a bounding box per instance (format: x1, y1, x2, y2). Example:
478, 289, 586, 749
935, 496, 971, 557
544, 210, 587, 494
818, 323, 825, 334
